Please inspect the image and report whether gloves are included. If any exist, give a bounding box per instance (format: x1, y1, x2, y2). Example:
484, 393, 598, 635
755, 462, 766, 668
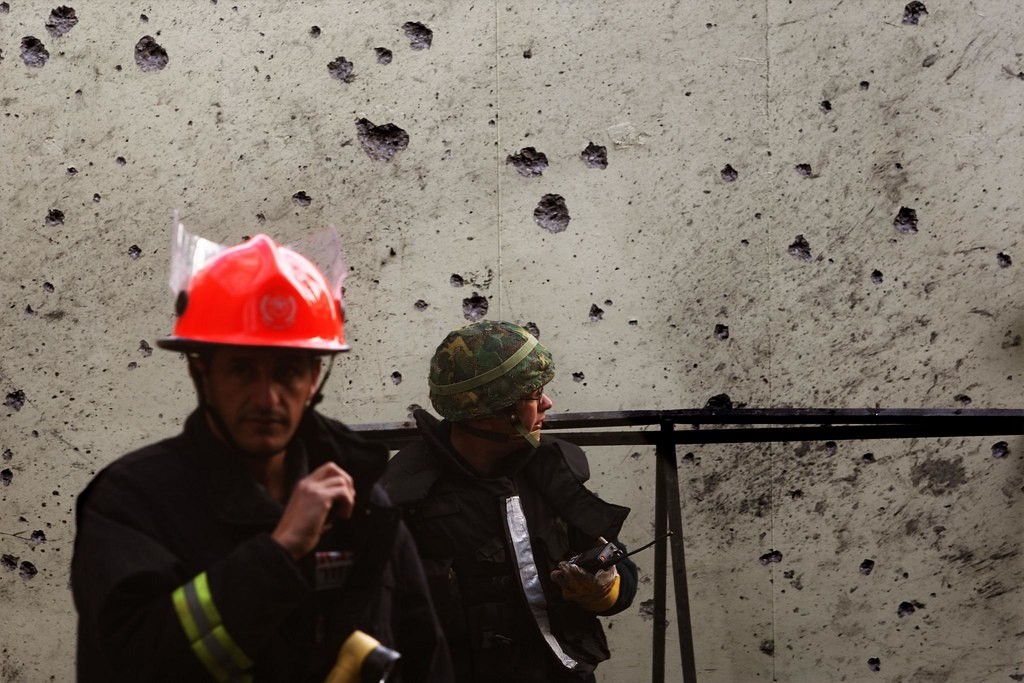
550, 536, 620, 612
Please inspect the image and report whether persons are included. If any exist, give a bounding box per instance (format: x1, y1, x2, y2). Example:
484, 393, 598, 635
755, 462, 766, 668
69, 234, 458, 683
380, 319, 637, 683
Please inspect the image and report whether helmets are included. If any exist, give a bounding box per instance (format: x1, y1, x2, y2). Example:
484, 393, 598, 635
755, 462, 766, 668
429, 320, 555, 421
158, 234, 352, 353
